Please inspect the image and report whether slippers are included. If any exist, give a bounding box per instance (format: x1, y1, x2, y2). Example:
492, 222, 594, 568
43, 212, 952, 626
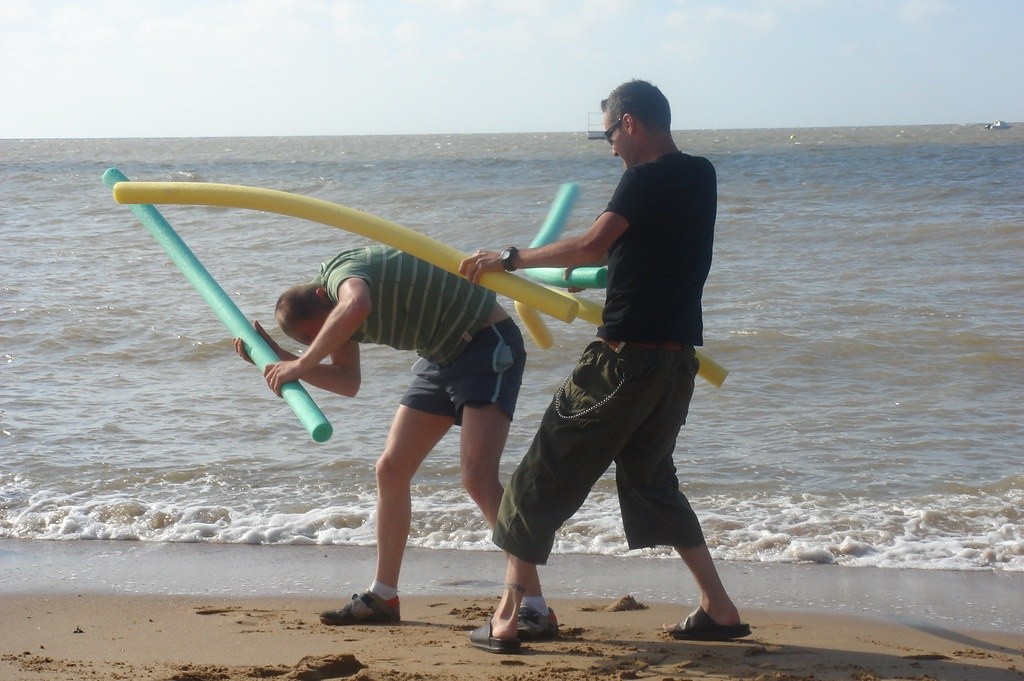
469, 614, 521, 655
669, 606, 752, 641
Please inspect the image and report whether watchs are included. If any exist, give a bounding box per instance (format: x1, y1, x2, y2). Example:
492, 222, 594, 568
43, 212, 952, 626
500, 247, 517, 272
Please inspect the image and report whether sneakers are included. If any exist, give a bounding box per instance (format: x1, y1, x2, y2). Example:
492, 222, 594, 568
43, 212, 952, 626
319, 591, 401, 625
517, 604, 558, 642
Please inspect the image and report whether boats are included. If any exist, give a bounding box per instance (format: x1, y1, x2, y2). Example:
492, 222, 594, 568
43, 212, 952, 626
985, 122, 1012, 130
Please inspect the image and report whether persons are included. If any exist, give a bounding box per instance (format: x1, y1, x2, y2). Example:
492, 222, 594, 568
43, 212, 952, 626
231, 238, 561, 643
459, 78, 754, 656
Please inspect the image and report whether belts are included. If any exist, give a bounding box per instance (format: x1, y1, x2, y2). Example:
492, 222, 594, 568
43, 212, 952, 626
603, 338, 690, 354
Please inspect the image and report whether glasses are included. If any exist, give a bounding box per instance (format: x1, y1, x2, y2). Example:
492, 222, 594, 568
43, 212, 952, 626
604, 119, 621, 145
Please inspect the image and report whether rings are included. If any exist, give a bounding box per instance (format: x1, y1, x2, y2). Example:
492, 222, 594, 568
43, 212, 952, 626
270, 379, 273, 382
